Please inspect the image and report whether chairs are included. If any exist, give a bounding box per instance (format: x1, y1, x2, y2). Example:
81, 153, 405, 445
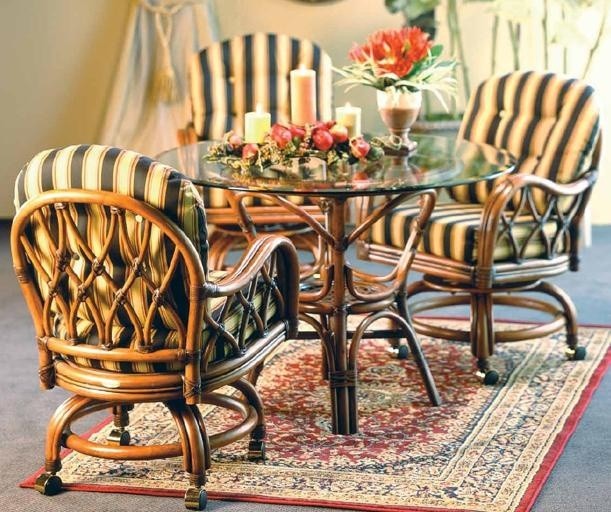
8, 142, 301, 509
187, 33, 332, 273
352, 64, 604, 387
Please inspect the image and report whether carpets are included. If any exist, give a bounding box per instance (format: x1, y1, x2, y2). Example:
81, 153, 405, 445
14, 312, 611, 512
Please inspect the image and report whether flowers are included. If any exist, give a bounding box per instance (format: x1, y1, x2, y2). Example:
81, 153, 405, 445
328, 28, 462, 113
197, 124, 388, 189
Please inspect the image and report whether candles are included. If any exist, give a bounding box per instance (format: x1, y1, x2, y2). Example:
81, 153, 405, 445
287, 62, 321, 127
333, 100, 366, 139
240, 100, 276, 147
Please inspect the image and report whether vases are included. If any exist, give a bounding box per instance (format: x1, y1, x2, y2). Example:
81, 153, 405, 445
371, 85, 426, 156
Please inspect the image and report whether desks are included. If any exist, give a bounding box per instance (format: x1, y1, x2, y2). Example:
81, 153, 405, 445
148, 131, 518, 437
